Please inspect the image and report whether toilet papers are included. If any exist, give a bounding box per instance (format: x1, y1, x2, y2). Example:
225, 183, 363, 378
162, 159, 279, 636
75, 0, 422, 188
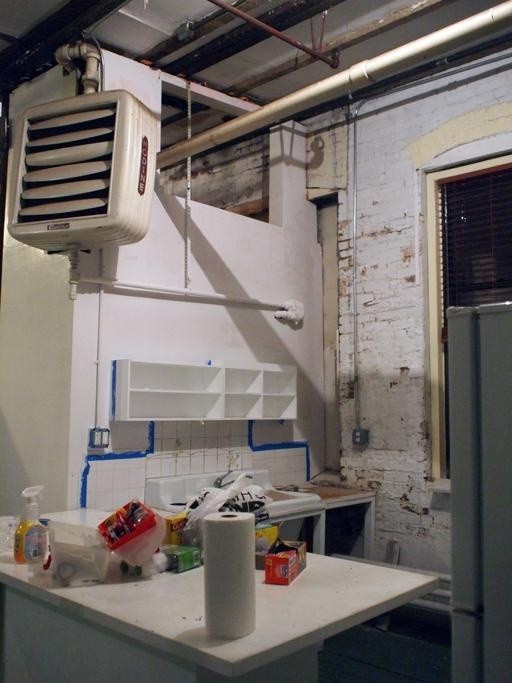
200, 511, 255, 641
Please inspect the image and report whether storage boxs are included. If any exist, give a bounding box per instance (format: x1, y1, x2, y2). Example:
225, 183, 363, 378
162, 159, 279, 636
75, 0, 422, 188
41, 508, 166, 580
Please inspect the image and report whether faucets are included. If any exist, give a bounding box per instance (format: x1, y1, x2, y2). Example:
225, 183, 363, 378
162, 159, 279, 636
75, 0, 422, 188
214, 470, 234, 488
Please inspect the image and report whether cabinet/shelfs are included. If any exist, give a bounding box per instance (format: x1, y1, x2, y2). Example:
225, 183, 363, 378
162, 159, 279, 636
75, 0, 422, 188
112, 357, 299, 422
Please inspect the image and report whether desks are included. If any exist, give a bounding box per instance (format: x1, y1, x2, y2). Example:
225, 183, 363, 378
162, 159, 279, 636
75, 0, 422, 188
0, 505, 438, 683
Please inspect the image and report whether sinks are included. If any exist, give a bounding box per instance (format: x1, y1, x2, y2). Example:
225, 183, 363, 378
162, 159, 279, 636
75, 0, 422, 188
164, 489, 322, 513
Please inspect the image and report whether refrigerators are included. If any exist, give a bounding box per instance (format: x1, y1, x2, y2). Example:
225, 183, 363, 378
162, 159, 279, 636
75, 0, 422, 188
446, 303, 512, 682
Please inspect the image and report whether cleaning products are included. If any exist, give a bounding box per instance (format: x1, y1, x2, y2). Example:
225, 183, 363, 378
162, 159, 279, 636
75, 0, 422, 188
14, 484, 49, 564
